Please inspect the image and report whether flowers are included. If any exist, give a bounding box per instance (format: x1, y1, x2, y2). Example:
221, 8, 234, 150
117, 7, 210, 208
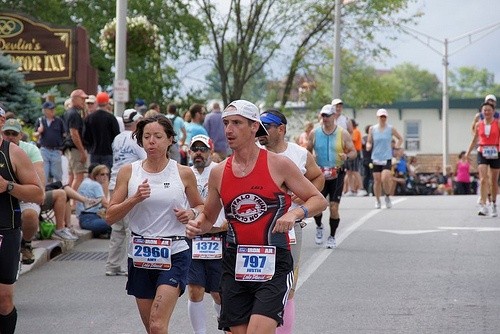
100, 16, 163, 60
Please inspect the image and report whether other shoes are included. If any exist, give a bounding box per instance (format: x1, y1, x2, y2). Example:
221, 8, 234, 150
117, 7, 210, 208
342, 190, 392, 209
83, 197, 103, 210
20, 242, 35, 264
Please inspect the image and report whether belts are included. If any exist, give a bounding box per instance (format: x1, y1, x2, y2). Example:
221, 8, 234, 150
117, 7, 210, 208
41, 144, 60, 150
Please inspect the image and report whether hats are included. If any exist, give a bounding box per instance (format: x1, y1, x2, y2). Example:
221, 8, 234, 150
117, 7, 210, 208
189, 134, 214, 149
134, 99, 145, 107
221, 99, 269, 137
71, 89, 109, 103
376, 108, 389, 117
484, 95, 497, 104
260, 112, 284, 125
331, 98, 344, 105
320, 104, 336, 115
122, 108, 143, 124
42, 102, 56, 109
1, 118, 22, 133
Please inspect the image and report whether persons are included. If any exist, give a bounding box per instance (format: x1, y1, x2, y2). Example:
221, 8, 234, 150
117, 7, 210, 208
341, 116, 481, 195
255, 108, 325, 333
105, 114, 205, 334
0, 107, 44, 334
184, 99, 328, 334
307, 104, 357, 248
103, 107, 147, 277
329, 98, 356, 207
366, 108, 405, 209
471, 93, 500, 204
464, 102, 500, 217
179, 133, 230, 334
1, 117, 44, 265
36, 86, 326, 241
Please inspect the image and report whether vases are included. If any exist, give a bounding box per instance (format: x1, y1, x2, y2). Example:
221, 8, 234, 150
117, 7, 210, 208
125, 52, 146, 66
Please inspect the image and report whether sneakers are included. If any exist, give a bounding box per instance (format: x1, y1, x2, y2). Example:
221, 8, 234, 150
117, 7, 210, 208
105, 269, 128, 276
326, 236, 336, 249
50, 227, 78, 241
65, 224, 82, 237
315, 223, 324, 245
477, 201, 498, 217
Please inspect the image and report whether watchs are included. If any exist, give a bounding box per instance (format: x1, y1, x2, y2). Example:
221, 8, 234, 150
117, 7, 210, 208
190, 207, 200, 218
6, 180, 14, 193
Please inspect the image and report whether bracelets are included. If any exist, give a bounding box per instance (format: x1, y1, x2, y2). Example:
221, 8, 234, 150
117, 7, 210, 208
299, 204, 309, 218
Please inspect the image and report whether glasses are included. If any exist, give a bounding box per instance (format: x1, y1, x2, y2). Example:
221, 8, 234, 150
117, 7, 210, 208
2, 130, 19, 137
85, 102, 95, 105
262, 122, 279, 130
190, 146, 210, 153
321, 113, 333, 118
100, 173, 109, 177
